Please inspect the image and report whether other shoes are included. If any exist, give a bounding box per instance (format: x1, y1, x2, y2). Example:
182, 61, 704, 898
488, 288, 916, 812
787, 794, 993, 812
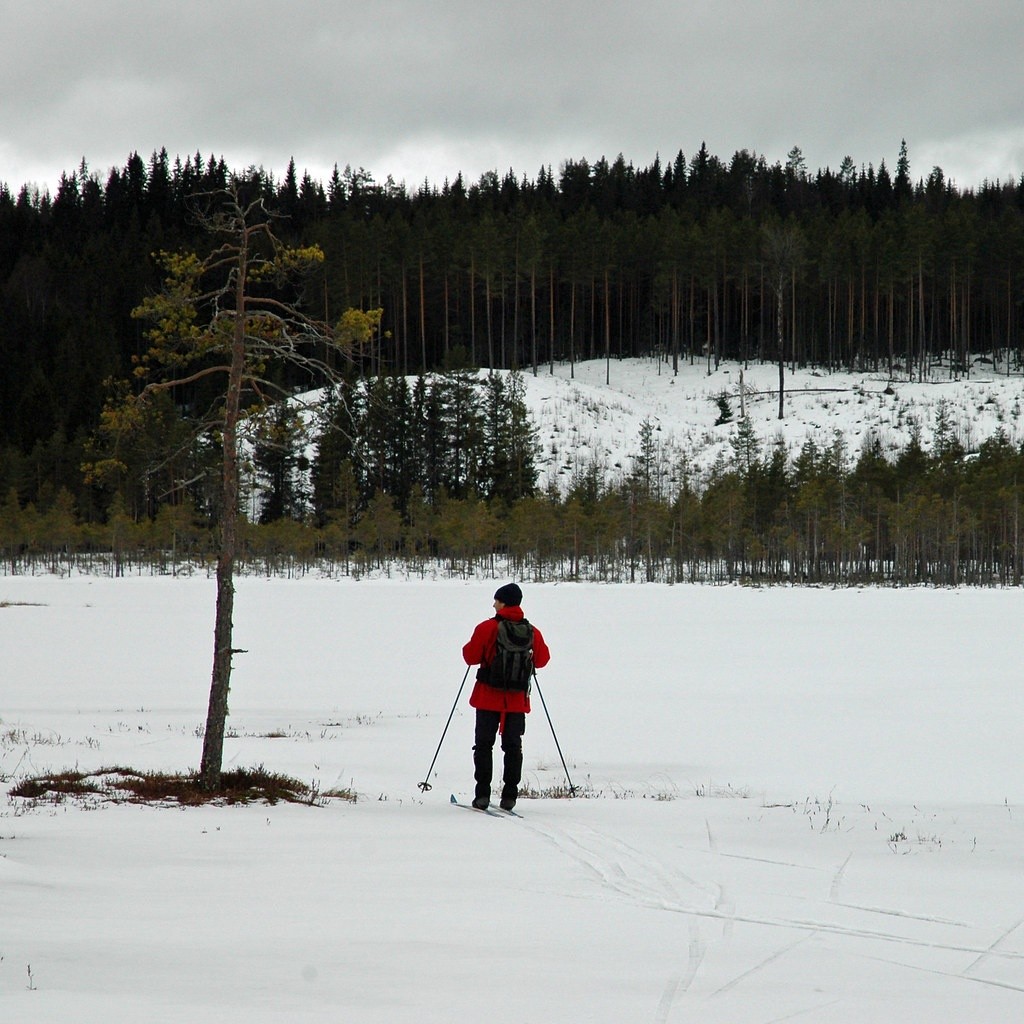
500, 798, 516, 810
472, 795, 489, 810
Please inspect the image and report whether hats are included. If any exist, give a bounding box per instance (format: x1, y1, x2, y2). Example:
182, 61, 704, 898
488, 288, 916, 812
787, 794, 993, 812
494, 583, 522, 607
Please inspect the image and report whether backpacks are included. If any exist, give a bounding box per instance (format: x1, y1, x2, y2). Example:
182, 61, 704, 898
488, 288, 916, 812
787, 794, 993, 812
476, 614, 534, 691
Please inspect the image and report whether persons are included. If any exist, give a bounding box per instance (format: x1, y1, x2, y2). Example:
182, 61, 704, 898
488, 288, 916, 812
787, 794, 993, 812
462, 582, 550, 811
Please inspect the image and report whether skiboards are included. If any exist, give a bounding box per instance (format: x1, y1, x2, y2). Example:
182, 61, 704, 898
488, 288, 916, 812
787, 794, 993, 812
450, 793, 527, 819
